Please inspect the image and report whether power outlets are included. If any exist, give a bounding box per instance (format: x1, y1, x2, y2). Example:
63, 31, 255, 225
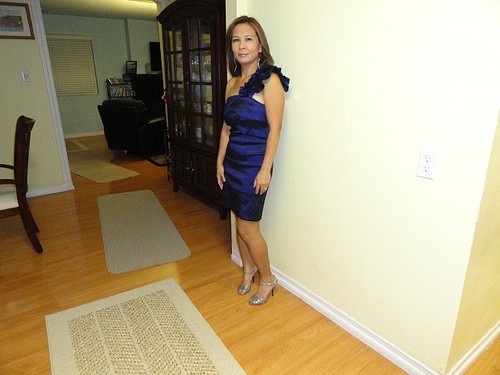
107, 79, 136, 100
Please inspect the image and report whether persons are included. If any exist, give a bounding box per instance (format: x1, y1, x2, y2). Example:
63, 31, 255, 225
215, 15, 291, 305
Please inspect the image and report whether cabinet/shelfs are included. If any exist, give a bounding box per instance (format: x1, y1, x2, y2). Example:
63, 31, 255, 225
156, 0, 231, 220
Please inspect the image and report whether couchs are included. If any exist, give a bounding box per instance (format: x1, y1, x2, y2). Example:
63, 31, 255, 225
98, 101, 166, 157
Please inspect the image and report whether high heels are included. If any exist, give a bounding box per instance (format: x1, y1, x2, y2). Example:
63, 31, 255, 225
238, 262, 258, 295
248, 275, 277, 305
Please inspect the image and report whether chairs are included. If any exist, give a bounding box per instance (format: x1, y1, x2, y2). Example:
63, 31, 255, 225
0, 116, 44, 255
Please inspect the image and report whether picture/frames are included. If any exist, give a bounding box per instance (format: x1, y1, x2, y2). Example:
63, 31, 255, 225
126, 61, 137, 74
0, 2, 35, 39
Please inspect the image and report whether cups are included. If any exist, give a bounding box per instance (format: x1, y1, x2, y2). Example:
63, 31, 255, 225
196, 128, 202, 138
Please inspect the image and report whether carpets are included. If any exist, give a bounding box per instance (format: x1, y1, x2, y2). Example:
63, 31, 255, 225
70, 158, 140, 184
97, 189, 191, 273
145, 153, 172, 167
45, 278, 246, 375
65, 139, 90, 153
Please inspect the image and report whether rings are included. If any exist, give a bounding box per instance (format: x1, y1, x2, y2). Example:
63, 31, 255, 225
264, 188, 267, 190
